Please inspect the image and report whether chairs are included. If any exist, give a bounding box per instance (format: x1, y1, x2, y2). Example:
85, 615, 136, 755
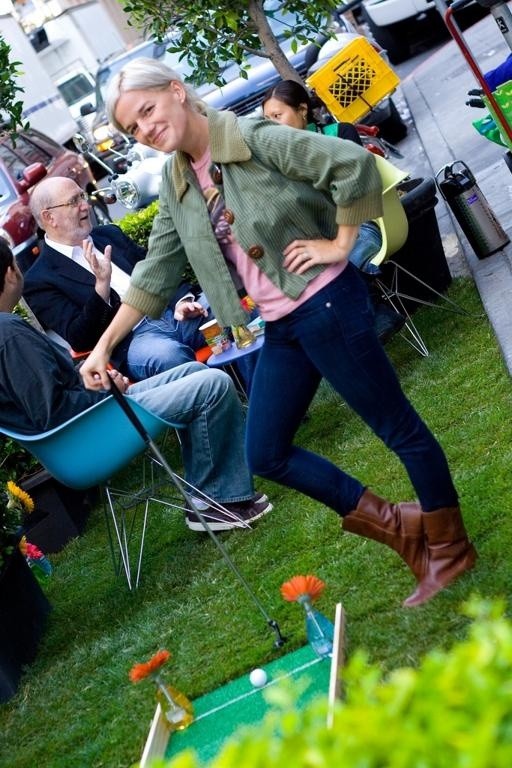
0, 393, 254, 593
369, 151, 489, 358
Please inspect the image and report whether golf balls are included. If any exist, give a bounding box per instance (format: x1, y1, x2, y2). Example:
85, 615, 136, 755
250, 668, 267, 686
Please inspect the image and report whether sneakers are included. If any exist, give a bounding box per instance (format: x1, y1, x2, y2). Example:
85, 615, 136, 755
376, 304, 404, 346
183, 495, 273, 532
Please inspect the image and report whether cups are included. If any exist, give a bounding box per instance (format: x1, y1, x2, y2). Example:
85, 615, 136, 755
198, 318, 224, 340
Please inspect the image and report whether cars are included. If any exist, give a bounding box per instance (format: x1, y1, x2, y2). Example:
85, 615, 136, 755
0, 0, 512, 270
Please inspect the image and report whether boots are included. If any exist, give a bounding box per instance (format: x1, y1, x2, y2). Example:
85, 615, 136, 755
402, 502, 475, 605
342, 489, 428, 580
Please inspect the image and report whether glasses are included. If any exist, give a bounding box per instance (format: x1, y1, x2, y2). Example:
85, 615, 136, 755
45, 193, 87, 211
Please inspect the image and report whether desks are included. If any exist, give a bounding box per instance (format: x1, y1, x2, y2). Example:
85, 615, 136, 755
206, 314, 266, 410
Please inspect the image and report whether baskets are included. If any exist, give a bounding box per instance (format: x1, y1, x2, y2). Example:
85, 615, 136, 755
305, 37, 399, 123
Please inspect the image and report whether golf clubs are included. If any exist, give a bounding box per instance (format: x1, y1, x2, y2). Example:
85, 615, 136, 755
104, 370, 287, 651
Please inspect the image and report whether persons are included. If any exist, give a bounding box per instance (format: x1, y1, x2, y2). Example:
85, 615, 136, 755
260, 79, 408, 348
1, 235, 275, 532
19, 175, 260, 399
78, 57, 477, 613
